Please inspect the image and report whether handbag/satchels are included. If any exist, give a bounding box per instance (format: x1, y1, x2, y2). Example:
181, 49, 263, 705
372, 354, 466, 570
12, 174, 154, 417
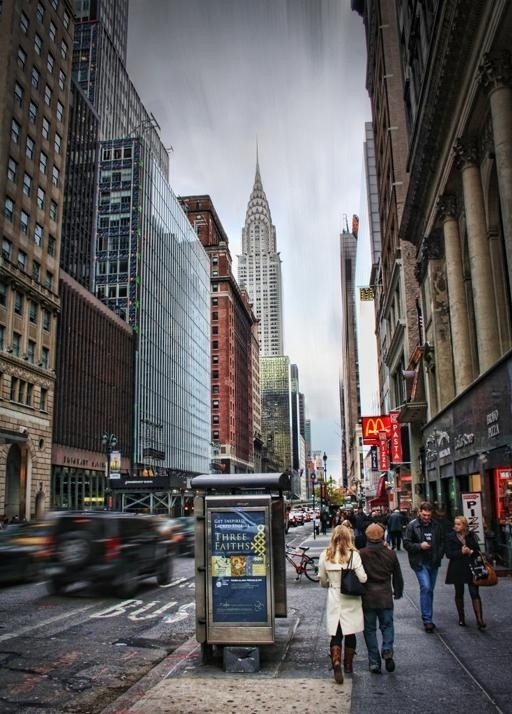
340, 550, 365, 596
471, 551, 498, 587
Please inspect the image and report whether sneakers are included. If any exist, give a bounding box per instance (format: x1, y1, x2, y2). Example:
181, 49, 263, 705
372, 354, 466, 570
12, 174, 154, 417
423, 621, 435, 633
369, 663, 382, 674
382, 652, 395, 672
391, 544, 397, 550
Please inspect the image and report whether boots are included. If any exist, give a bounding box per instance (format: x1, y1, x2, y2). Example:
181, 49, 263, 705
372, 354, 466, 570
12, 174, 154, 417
329, 645, 344, 683
454, 596, 466, 627
471, 598, 486, 630
344, 646, 355, 674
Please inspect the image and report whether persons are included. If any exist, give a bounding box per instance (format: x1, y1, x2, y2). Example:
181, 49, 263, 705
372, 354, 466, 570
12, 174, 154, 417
402, 500, 446, 632
314, 495, 467, 560
443, 515, 488, 631
318, 525, 367, 683
356, 524, 405, 676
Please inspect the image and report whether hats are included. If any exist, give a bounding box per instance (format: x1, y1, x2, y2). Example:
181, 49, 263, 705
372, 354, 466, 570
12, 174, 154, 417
365, 522, 385, 540
393, 509, 400, 513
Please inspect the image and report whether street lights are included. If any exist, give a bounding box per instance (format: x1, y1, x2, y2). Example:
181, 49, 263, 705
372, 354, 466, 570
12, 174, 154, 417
101, 428, 120, 510
323, 451, 328, 483
